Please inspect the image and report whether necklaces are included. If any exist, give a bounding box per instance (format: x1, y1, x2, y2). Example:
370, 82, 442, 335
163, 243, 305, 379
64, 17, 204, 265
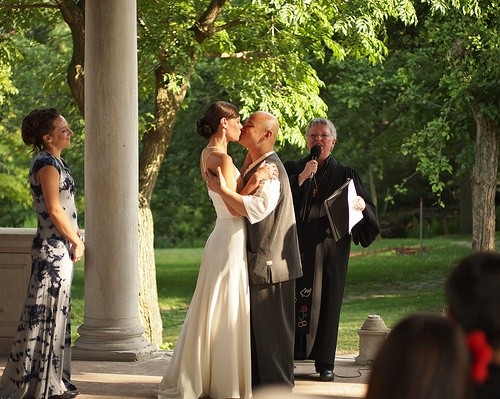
312, 170, 326, 198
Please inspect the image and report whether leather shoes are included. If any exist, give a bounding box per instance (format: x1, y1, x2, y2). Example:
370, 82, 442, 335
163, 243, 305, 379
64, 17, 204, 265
320, 371, 334, 380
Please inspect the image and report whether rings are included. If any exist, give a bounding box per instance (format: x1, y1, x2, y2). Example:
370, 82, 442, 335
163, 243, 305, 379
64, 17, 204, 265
273, 171, 275, 175
206, 181, 208, 183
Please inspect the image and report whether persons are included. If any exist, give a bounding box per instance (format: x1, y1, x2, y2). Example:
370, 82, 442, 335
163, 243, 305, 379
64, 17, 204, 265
0, 107, 84, 399
282, 117, 380, 381
197, 101, 303, 399
366, 251, 500, 399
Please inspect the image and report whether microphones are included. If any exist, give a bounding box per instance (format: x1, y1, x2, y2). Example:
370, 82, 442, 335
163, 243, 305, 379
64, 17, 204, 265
306, 145, 321, 189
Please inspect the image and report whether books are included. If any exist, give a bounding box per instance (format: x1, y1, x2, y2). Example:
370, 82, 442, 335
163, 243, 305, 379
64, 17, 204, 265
323, 179, 363, 242
